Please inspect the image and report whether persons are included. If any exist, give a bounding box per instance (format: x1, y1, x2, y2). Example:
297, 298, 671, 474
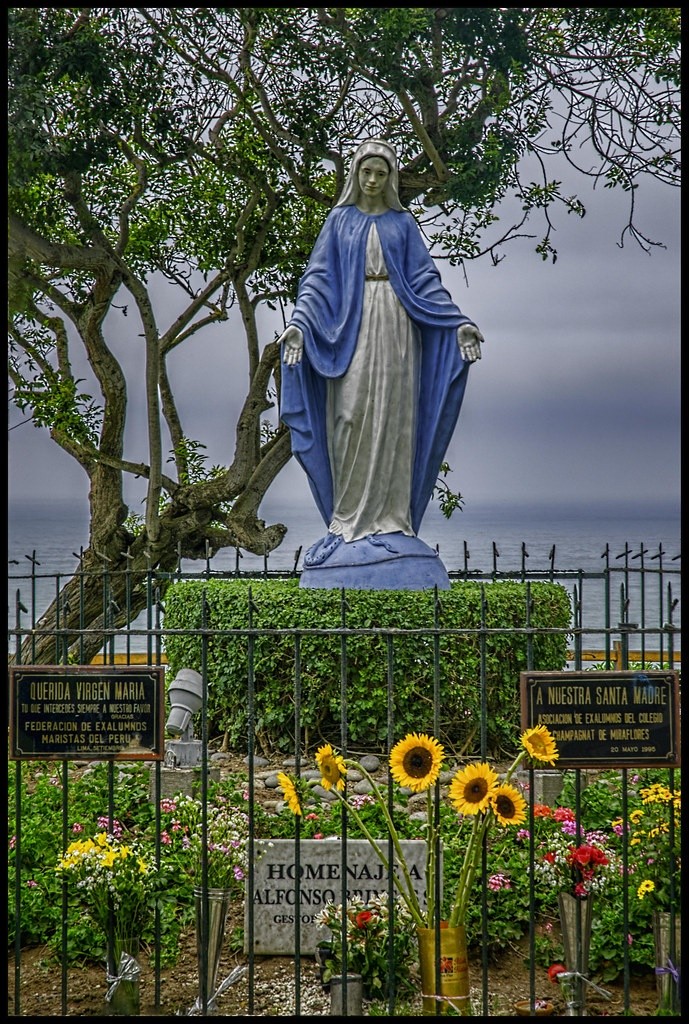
271, 136, 488, 540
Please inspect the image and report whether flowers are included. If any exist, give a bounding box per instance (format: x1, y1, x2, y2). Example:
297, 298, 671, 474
547, 962, 576, 1016
609, 782, 681, 911
516, 803, 623, 900
277, 722, 560, 928
54, 832, 160, 936
314, 893, 426, 993
160, 795, 250, 886
72, 817, 136, 839
27, 880, 38, 889
9, 836, 16, 852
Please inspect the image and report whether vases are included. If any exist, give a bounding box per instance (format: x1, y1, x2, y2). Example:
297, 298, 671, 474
102, 938, 144, 1016
416, 926, 475, 1015
556, 892, 595, 1016
651, 912, 681, 1013
191, 886, 229, 1012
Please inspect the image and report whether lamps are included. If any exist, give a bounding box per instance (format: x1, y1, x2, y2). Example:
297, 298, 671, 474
162, 669, 209, 770
